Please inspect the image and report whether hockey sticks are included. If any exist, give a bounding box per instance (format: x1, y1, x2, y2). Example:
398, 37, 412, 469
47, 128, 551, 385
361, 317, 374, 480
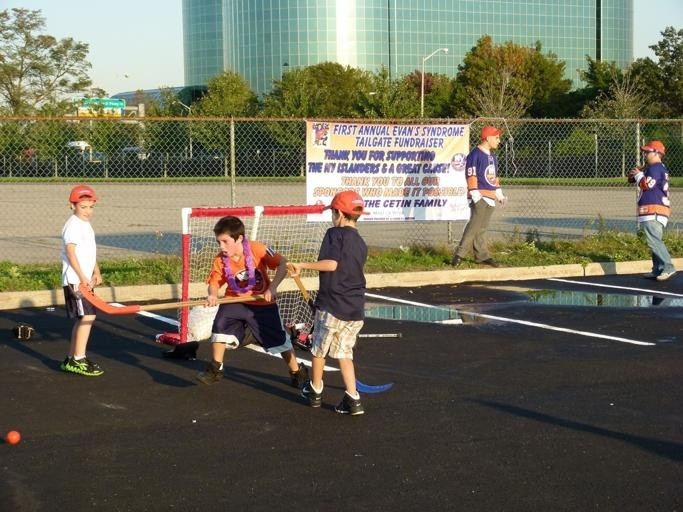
286, 262, 395, 394
66, 272, 97, 300
79, 283, 277, 315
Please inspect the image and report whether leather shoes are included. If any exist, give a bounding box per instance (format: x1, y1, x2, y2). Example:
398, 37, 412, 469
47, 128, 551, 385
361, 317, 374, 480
480, 256, 499, 267
452, 254, 461, 269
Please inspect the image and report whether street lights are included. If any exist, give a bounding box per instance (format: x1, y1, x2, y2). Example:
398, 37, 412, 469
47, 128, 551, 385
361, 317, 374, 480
420, 48, 448, 117
419, 46, 449, 124
175, 100, 193, 158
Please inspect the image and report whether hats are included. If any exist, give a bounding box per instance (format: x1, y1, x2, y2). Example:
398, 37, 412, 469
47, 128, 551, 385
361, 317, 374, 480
323, 191, 366, 218
69, 185, 98, 202
481, 125, 503, 140
639, 139, 665, 154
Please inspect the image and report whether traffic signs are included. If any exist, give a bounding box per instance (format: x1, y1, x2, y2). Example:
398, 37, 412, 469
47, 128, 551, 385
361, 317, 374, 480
81, 96, 124, 109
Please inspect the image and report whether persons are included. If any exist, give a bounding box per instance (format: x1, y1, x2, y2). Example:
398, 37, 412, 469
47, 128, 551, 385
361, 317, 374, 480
194, 216, 312, 394
627, 141, 677, 281
299, 190, 370, 417
60, 185, 105, 376
452, 126, 507, 268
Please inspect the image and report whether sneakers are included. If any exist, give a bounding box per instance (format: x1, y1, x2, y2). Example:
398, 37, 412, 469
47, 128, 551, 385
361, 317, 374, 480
67, 354, 105, 377
290, 363, 312, 388
299, 382, 324, 409
657, 269, 679, 282
333, 390, 364, 417
196, 361, 223, 386
